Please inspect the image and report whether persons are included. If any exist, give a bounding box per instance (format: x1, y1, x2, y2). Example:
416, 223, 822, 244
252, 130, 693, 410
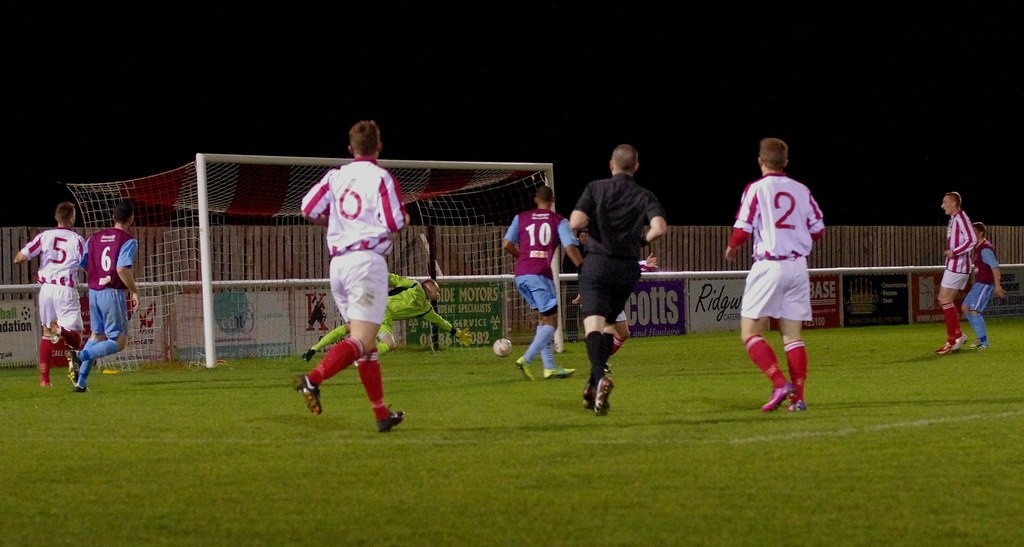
302, 272, 471, 361
562, 230, 586, 342
568, 144, 666, 417
935, 192, 977, 354
65, 203, 140, 391
573, 252, 657, 373
503, 186, 582, 379
960, 222, 1006, 349
13, 202, 86, 386
725, 138, 826, 412
291, 121, 410, 431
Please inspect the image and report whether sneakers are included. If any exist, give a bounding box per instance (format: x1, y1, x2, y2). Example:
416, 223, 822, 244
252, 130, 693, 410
302, 348, 316, 362
761, 381, 797, 412
935, 338, 961, 355
954, 332, 969, 348
377, 411, 405, 431
50, 320, 62, 345
582, 385, 596, 409
543, 366, 575, 380
593, 374, 614, 417
515, 356, 536, 382
786, 400, 807, 413
72, 382, 90, 393
974, 343, 990, 350
296, 373, 322, 415
603, 363, 613, 374
65, 349, 82, 384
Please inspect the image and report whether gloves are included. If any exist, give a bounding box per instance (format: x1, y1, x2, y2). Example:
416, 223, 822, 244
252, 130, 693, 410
455, 327, 473, 347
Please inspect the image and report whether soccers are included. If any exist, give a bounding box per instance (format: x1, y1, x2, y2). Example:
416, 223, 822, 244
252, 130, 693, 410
493, 337, 513, 357
